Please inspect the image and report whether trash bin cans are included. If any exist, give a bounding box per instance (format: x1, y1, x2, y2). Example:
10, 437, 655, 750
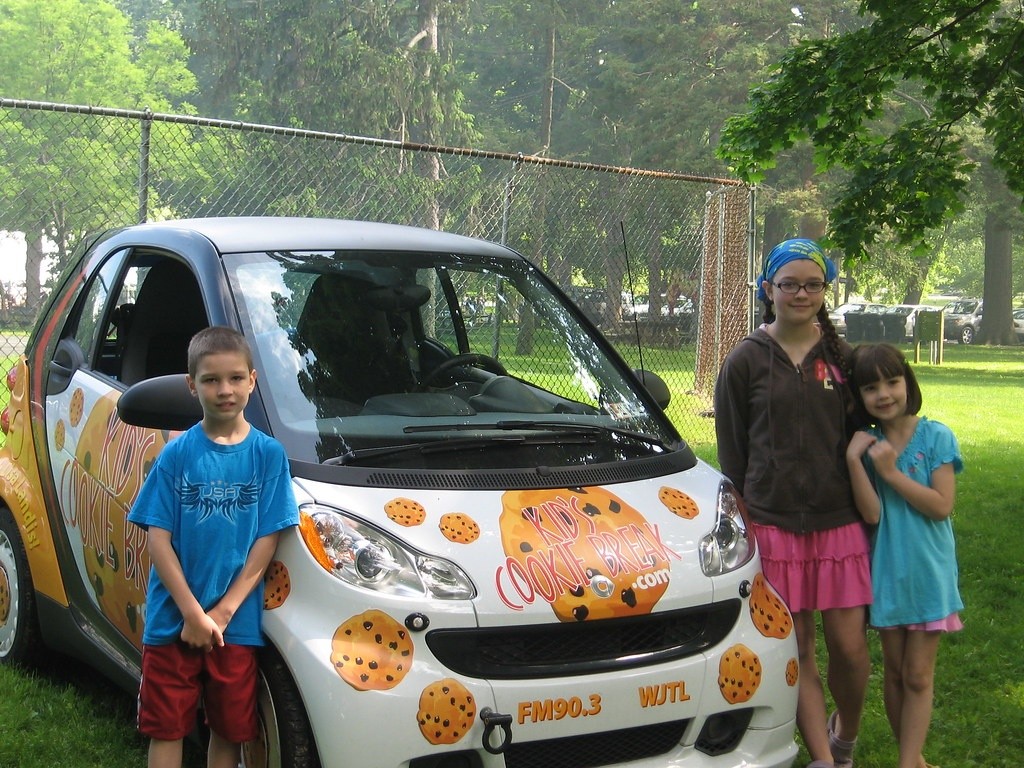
843, 313, 864, 343
882, 313, 908, 343
862, 313, 885, 342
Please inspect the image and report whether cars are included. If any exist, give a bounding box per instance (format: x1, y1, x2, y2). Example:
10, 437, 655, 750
0, 216, 799, 768
433, 287, 1024, 342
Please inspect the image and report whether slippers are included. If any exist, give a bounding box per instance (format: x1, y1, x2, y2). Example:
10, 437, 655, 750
806, 760, 833, 768
827, 708, 857, 768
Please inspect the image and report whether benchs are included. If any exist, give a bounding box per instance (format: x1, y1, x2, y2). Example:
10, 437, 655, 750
604, 332, 642, 341
441, 327, 480, 332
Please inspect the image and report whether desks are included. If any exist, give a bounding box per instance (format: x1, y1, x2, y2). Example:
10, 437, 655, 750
618, 320, 683, 346
434, 316, 487, 332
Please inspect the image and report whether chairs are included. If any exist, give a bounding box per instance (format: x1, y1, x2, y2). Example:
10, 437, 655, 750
118, 263, 209, 387
294, 270, 426, 400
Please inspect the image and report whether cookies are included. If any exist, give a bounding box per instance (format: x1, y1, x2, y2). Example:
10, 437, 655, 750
54, 387, 85, 452
262, 487, 800, 745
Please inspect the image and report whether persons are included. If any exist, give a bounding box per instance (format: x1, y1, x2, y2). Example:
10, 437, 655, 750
714, 238, 875, 767
845, 342, 965, 768
124, 325, 299, 768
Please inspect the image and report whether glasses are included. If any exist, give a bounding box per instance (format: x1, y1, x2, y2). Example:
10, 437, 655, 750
768, 279, 829, 294
